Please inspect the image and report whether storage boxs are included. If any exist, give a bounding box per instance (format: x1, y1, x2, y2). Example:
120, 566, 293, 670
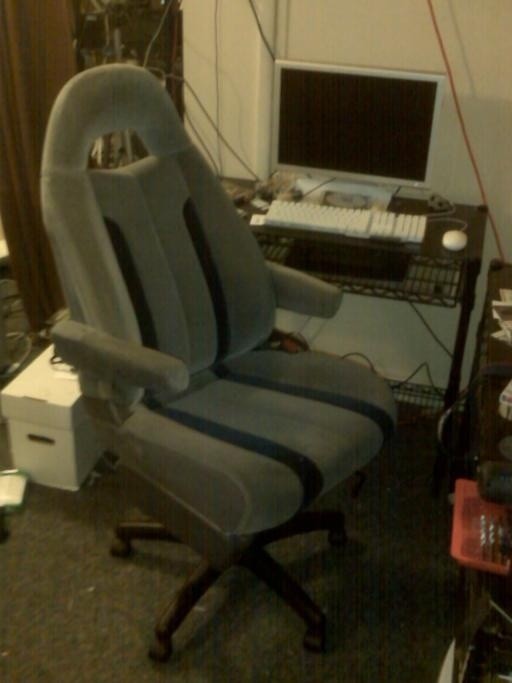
0, 344, 106, 493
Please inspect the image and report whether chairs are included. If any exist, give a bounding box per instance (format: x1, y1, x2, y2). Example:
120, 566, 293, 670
38, 61, 402, 665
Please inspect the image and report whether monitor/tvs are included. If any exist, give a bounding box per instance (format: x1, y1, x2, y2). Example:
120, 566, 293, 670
269, 58, 447, 213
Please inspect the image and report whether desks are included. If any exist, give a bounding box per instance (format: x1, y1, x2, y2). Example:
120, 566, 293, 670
219, 175, 488, 425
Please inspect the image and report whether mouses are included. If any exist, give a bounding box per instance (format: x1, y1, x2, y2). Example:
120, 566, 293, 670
441, 229, 469, 251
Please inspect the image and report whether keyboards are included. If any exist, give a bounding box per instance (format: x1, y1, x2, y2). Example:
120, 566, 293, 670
264, 197, 430, 246
